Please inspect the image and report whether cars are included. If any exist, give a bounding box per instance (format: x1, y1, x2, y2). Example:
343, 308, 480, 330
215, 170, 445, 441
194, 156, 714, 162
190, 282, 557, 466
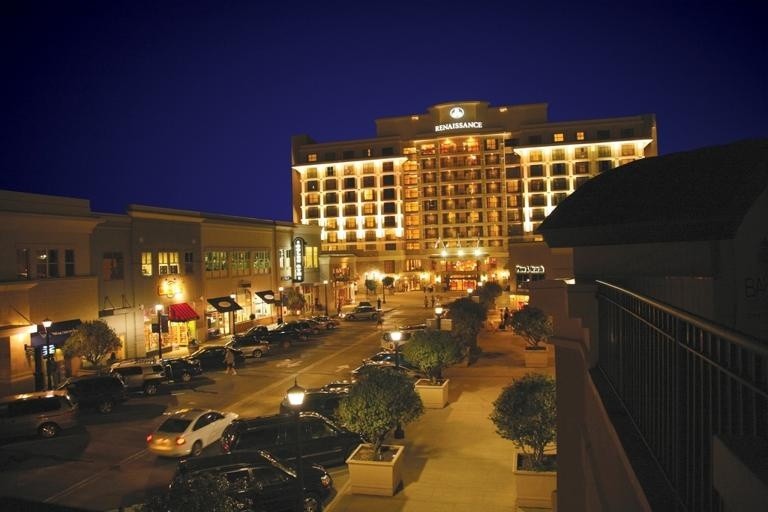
339, 305, 384, 321
278, 324, 427, 416
158, 314, 341, 383
146, 407, 239, 457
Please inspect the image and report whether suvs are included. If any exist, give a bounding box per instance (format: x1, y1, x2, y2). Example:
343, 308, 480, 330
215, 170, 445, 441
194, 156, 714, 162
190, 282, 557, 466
220, 410, 368, 468
107, 361, 167, 396
167, 449, 333, 512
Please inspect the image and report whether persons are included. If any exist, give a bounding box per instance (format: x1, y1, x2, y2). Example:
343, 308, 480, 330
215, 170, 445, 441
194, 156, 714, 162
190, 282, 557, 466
222, 348, 232, 374
230, 350, 238, 376
376, 297, 382, 309
503, 306, 510, 326
499, 308, 503, 326
186, 328, 192, 341
375, 310, 384, 330
335, 302, 341, 316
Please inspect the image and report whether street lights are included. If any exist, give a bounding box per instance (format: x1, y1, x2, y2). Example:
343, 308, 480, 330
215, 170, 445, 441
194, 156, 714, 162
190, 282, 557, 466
230, 292, 236, 340
467, 274, 495, 303
278, 286, 284, 322
365, 271, 387, 304
285, 375, 307, 512
42, 316, 53, 390
434, 302, 444, 377
390, 325, 404, 439
323, 280, 329, 316
156, 304, 163, 359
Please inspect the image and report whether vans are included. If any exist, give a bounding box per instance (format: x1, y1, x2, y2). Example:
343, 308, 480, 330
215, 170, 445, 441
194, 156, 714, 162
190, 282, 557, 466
0, 388, 80, 439
56, 372, 128, 415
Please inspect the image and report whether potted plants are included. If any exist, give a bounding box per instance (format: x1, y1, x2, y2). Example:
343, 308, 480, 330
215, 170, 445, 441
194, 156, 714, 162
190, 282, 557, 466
439, 281, 501, 331
507, 303, 556, 370
396, 323, 474, 412
334, 359, 425, 498
443, 295, 483, 369
486, 371, 563, 510
59, 319, 122, 377
